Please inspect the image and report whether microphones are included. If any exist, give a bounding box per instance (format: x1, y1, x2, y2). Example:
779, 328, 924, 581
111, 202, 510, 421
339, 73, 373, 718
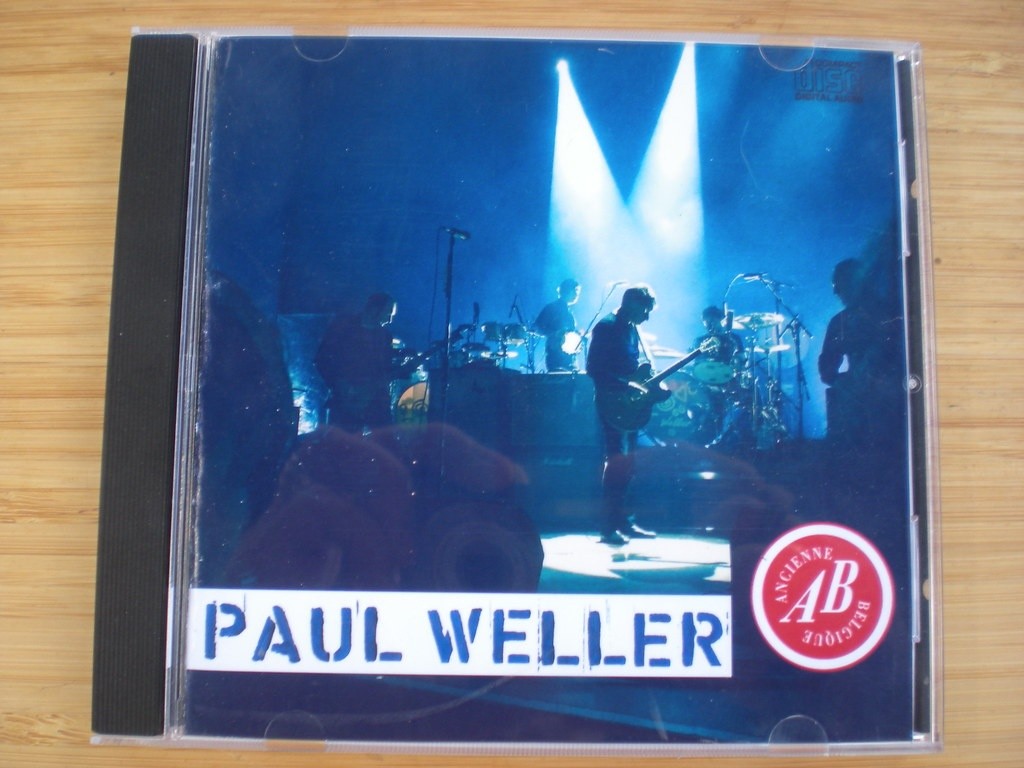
509, 293, 517, 318
764, 279, 787, 288
474, 302, 479, 324
443, 226, 471, 240
744, 272, 767, 280
615, 280, 627, 285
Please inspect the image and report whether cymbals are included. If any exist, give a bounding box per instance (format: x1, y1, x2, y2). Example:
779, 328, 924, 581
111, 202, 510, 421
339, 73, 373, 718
720, 311, 785, 330
743, 343, 790, 355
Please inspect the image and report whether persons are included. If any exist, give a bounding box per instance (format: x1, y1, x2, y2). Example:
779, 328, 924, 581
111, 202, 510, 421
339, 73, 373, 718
817, 259, 873, 389
587, 287, 670, 545
530, 278, 580, 375
315, 289, 413, 435
689, 303, 745, 400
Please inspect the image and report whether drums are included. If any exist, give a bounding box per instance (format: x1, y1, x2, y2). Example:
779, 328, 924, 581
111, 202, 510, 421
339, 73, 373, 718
638, 369, 726, 447
691, 332, 738, 386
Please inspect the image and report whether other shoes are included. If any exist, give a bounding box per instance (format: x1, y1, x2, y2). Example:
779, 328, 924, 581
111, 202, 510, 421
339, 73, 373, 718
628, 524, 656, 538
600, 530, 629, 546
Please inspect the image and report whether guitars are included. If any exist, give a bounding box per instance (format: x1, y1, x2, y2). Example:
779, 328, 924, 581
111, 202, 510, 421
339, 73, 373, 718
322, 323, 478, 423
592, 336, 722, 433
